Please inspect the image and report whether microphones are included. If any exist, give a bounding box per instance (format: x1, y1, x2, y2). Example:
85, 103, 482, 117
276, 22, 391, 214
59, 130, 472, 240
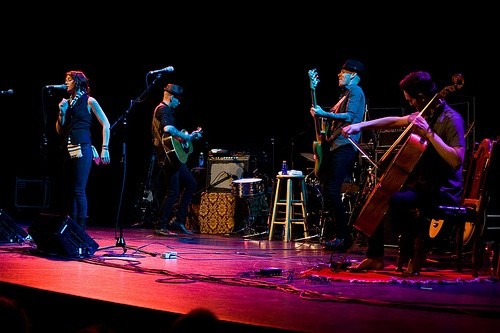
1, 89, 13, 94
45, 84, 66, 89
148, 66, 174, 74
225, 172, 238, 180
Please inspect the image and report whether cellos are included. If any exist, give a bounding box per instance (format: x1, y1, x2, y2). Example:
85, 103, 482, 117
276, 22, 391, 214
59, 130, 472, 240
340, 73, 466, 263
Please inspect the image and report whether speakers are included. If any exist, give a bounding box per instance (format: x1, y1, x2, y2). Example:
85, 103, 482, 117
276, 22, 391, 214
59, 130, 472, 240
205, 155, 251, 191
27, 213, 99, 260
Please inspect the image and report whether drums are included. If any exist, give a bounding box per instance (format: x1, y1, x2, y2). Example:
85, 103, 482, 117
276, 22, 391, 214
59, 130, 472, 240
376, 128, 408, 148
358, 143, 374, 156
229, 178, 265, 198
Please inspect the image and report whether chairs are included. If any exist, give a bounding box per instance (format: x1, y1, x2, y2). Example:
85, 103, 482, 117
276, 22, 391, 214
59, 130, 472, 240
398, 137, 493, 272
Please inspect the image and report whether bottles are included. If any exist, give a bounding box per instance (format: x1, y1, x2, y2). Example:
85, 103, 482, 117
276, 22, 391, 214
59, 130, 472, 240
282, 161, 287, 175
198, 152, 205, 167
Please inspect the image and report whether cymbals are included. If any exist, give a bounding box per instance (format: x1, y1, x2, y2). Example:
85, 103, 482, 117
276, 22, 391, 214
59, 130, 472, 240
300, 152, 315, 162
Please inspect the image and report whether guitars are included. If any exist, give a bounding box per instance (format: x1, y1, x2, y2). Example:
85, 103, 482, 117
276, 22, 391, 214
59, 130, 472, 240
161, 128, 204, 170
303, 63, 330, 180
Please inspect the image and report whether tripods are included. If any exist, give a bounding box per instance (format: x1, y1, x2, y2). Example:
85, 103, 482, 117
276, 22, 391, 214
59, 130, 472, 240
99, 72, 161, 257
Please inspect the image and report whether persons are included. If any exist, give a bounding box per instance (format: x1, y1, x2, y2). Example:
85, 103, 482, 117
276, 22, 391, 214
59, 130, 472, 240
151, 83, 202, 237
56, 70, 110, 231
309, 59, 365, 251
342, 72, 466, 277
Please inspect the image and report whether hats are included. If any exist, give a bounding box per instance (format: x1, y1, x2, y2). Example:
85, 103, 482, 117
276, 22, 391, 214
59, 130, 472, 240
164, 83, 184, 95
342, 58, 366, 74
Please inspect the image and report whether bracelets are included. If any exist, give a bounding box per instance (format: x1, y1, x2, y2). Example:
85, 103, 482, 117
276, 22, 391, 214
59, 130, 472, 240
101, 144, 109, 150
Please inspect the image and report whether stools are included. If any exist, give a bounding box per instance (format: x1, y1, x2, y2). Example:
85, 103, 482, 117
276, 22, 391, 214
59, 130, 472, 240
269, 175, 308, 243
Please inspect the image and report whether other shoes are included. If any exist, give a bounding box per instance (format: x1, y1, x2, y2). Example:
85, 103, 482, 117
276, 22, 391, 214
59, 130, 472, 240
348, 256, 384, 271
171, 221, 193, 235
153, 227, 178, 236
